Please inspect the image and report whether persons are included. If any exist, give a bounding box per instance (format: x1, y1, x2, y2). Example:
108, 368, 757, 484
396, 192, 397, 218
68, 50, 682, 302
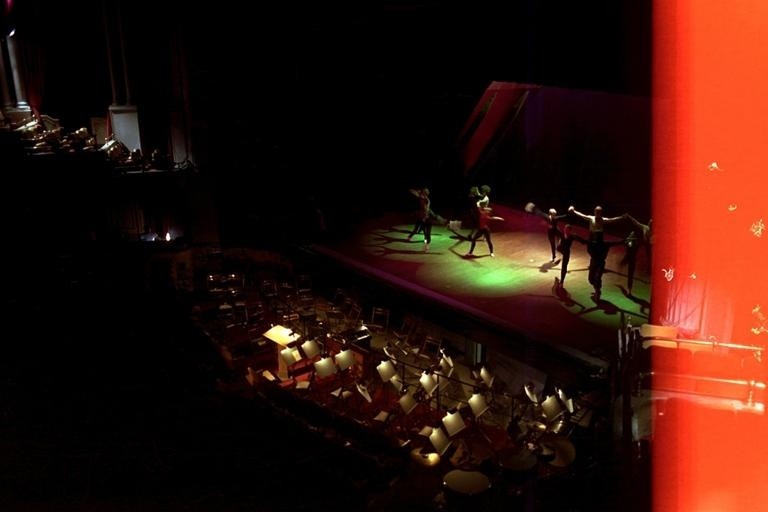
407, 185, 504, 257
525, 202, 651, 302
